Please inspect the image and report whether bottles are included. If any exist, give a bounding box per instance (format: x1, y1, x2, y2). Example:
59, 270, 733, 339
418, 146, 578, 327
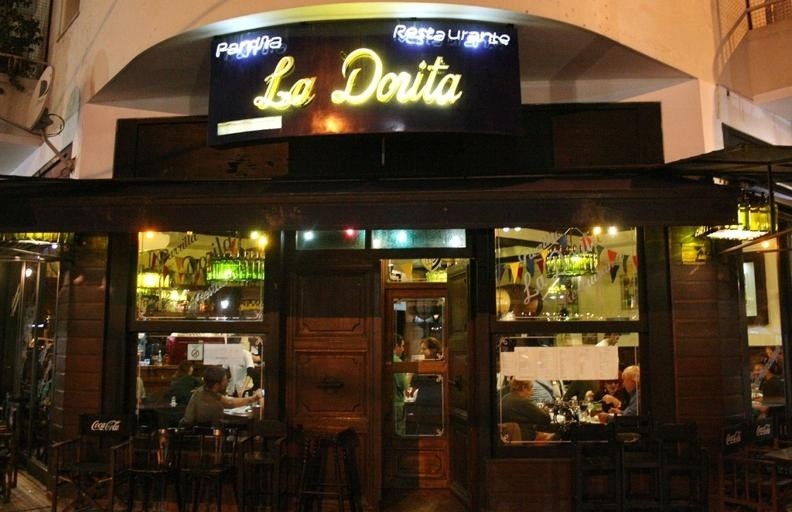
545, 244, 598, 277
707, 191, 780, 236
210, 248, 266, 281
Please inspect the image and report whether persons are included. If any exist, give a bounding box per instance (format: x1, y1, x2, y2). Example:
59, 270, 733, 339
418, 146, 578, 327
222, 336, 254, 397
393, 333, 415, 436
496, 332, 639, 442
179, 365, 262, 429
752, 360, 787, 450
410, 337, 443, 436
161, 360, 203, 428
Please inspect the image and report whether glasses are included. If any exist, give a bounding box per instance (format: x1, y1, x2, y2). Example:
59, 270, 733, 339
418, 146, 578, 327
606, 379, 620, 385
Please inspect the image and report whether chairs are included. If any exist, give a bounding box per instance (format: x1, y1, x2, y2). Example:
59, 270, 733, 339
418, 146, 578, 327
570, 407, 791, 512
